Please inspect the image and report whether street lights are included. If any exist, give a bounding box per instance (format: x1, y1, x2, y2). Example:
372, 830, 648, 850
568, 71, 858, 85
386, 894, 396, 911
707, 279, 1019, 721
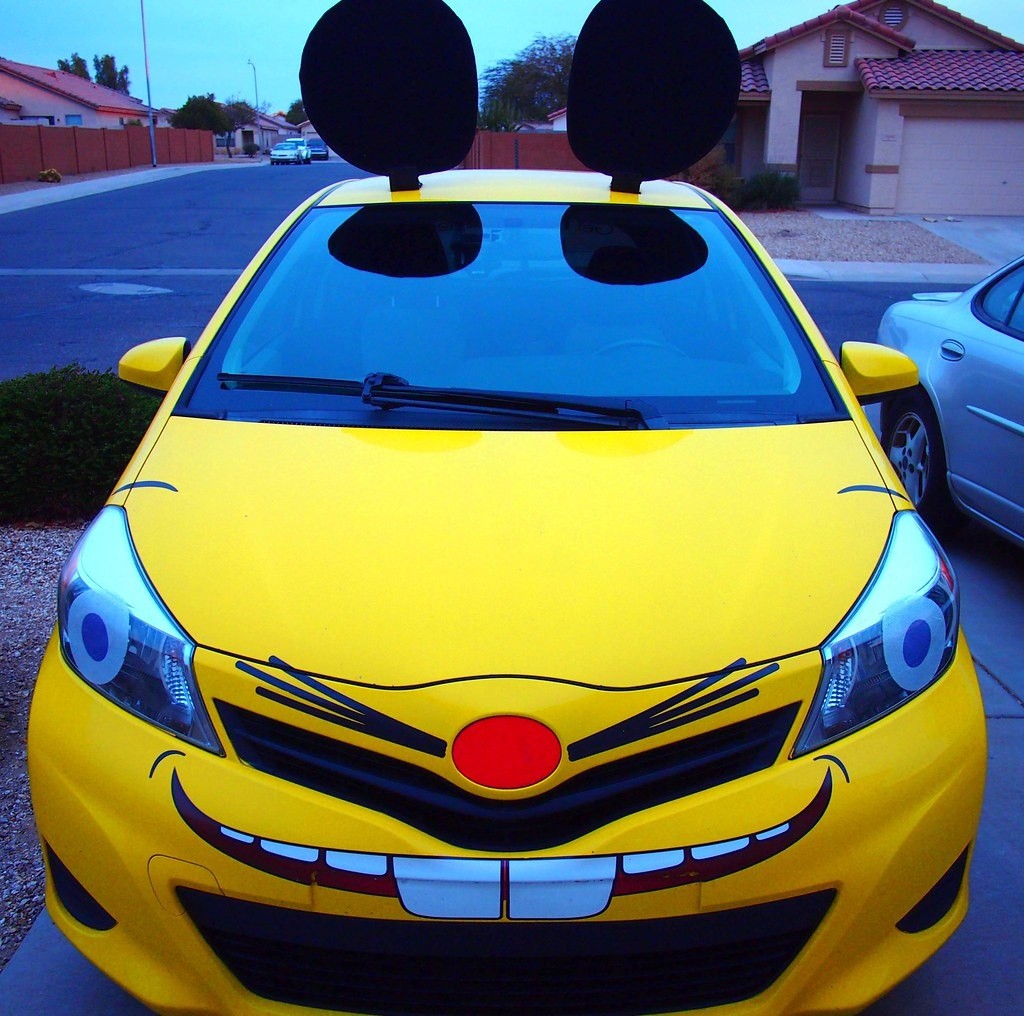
247, 63, 263, 161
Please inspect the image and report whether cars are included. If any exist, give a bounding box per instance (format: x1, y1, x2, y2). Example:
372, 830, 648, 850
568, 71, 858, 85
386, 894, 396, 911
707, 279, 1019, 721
269, 143, 303, 165
307, 138, 329, 160
286, 138, 312, 164
878, 255, 1023, 549
29, 172, 987, 1016
